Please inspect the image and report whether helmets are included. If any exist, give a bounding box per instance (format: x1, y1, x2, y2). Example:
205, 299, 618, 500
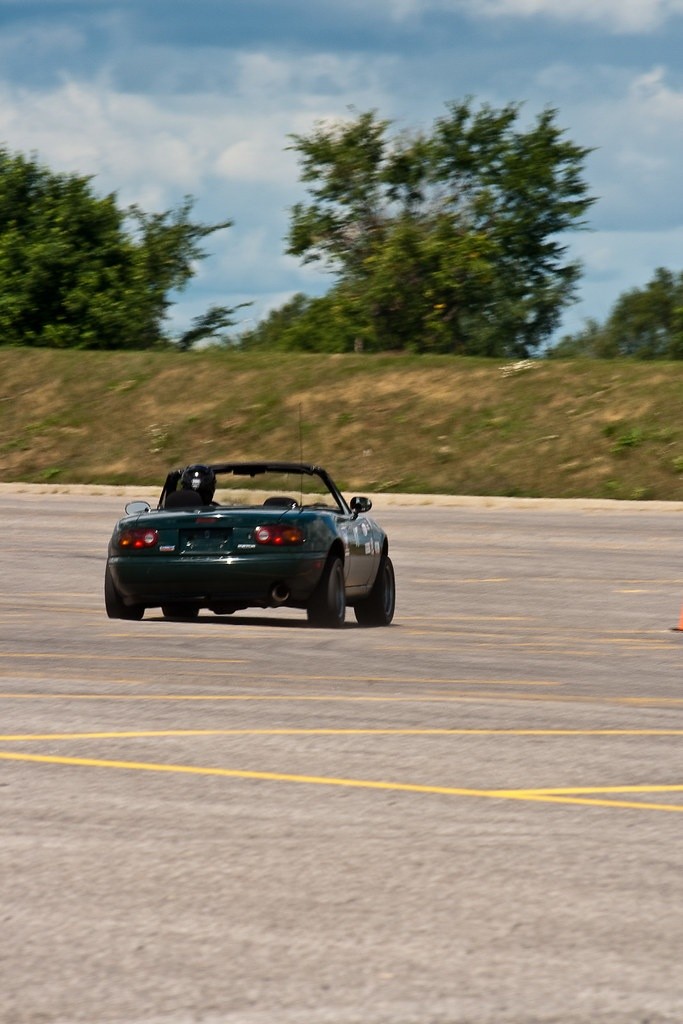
180, 465, 216, 502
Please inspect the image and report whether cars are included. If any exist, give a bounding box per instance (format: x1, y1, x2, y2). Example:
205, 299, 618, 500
104, 462, 396, 626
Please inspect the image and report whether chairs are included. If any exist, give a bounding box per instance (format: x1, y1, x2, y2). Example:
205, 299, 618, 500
165, 490, 203, 508
263, 497, 298, 507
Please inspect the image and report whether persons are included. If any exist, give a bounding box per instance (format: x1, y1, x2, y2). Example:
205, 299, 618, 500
181, 464, 217, 506
